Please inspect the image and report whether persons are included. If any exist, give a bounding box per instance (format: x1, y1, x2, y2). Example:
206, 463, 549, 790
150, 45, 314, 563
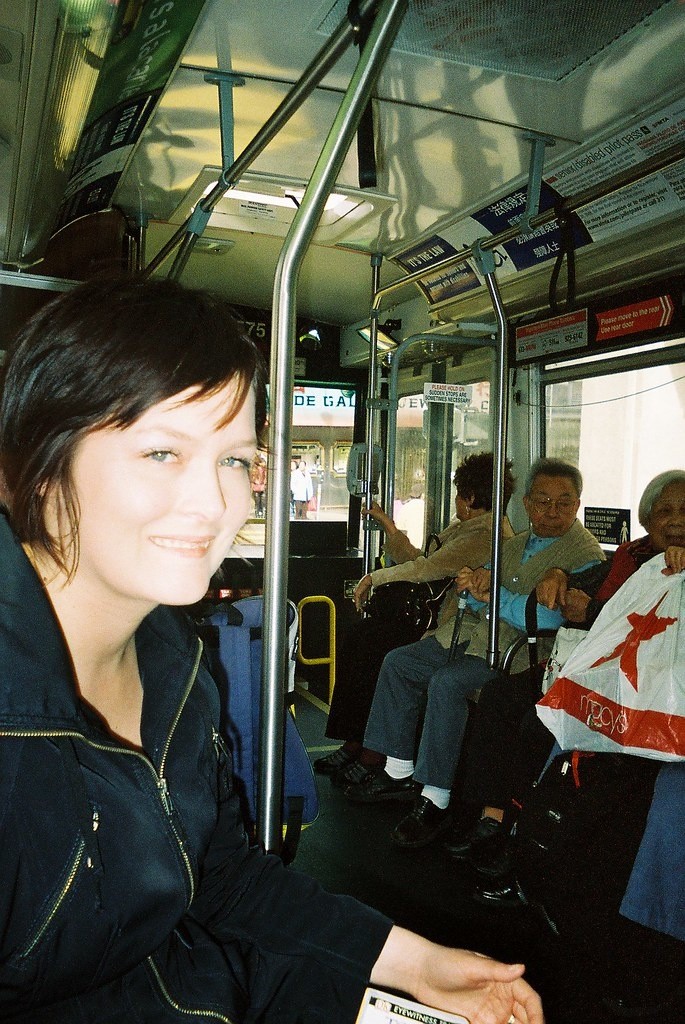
290, 461, 313, 520
1, 273, 544, 1024
253, 458, 267, 518
315, 450, 685, 1024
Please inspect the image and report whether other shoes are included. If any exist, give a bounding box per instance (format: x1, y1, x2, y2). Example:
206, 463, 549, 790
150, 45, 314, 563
313, 749, 357, 775
330, 761, 369, 786
470, 805, 521, 876
449, 830, 474, 853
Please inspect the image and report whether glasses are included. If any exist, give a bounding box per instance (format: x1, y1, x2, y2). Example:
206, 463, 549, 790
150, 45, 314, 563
528, 495, 577, 514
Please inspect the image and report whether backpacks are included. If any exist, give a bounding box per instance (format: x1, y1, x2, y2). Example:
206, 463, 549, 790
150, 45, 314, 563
201, 595, 321, 862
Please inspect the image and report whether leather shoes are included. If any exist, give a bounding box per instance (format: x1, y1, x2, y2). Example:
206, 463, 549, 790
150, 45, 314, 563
389, 796, 452, 848
344, 767, 421, 802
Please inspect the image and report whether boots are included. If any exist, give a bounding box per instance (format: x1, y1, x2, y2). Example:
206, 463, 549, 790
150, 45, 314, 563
475, 803, 577, 907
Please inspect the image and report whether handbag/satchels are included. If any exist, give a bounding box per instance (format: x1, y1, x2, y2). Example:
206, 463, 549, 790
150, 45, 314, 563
535, 552, 685, 763
361, 532, 457, 633
